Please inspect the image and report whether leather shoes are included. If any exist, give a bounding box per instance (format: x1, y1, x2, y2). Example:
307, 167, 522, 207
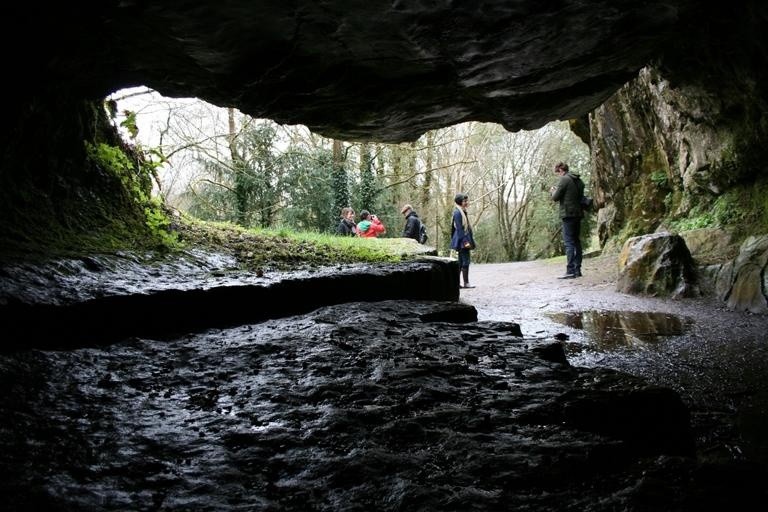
557, 271, 582, 279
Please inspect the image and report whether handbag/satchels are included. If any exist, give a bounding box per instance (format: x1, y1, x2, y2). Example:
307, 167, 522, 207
464, 240, 476, 250
581, 196, 593, 210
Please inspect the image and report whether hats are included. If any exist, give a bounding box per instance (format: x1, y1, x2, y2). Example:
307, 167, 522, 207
401, 204, 413, 214
454, 194, 465, 201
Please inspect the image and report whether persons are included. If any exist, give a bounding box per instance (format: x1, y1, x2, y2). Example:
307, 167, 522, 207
355, 209, 386, 240
448, 193, 477, 289
401, 205, 428, 245
550, 161, 586, 279
335, 207, 356, 237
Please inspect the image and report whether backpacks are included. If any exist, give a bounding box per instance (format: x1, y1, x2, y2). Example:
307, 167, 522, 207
417, 218, 428, 244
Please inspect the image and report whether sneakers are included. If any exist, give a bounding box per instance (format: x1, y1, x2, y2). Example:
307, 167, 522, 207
459, 282, 475, 289
